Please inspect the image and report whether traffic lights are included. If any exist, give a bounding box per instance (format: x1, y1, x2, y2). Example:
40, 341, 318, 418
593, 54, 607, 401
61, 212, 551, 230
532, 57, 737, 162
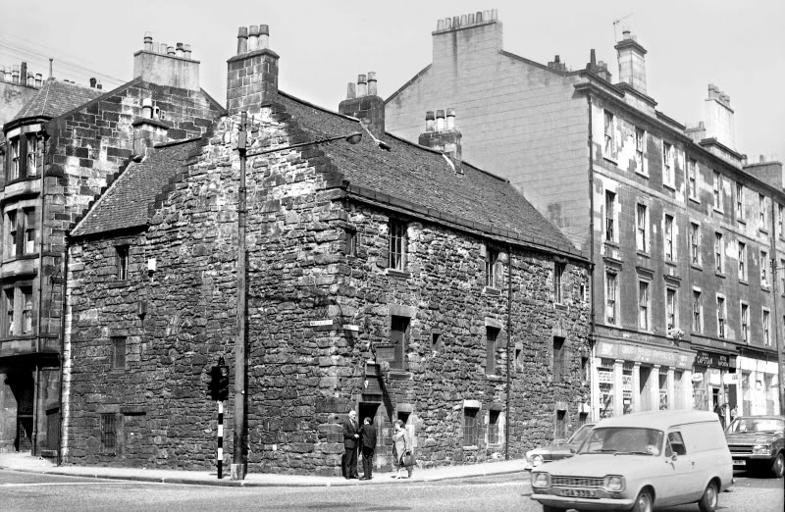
205, 368, 215, 396
218, 366, 229, 399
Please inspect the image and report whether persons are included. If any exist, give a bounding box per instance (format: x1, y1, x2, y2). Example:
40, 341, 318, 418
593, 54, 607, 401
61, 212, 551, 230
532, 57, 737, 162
731, 405, 738, 422
718, 404, 726, 430
357, 416, 378, 481
390, 420, 415, 480
342, 409, 361, 481
714, 404, 721, 416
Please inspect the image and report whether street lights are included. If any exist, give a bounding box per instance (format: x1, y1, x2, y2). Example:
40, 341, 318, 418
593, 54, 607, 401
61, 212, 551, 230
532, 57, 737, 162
229, 110, 362, 483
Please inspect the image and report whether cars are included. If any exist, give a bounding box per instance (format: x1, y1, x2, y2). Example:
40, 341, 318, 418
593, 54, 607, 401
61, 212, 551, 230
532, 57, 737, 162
526, 409, 785, 512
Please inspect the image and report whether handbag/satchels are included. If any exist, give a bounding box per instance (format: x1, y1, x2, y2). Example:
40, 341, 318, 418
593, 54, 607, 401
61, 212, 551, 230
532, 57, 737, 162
400, 454, 417, 467
